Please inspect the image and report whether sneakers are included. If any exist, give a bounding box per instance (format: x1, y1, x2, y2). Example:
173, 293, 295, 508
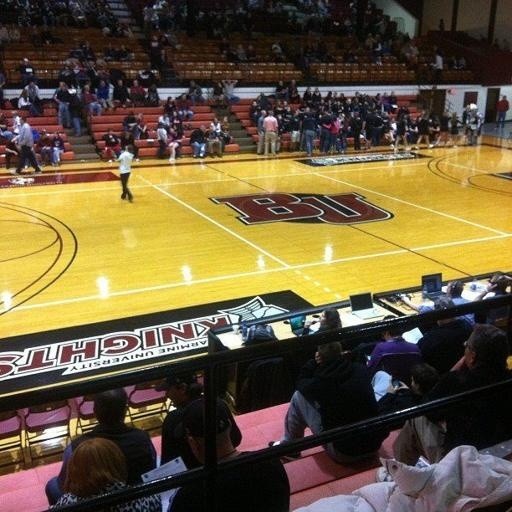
192, 152, 223, 158
268, 441, 301, 461
158, 156, 180, 162
42, 161, 62, 167
393, 142, 457, 152
121, 193, 133, 201
257, 146, 371, 157
108, 159, 119, 163
134, 157, 140, 161
16, 167, 41, 174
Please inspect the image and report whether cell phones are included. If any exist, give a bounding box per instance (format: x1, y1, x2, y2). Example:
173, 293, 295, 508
392, 377, 400, 387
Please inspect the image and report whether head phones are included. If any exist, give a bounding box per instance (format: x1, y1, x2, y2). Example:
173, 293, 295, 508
492, 275, 505, 286
449, 280, 459, 294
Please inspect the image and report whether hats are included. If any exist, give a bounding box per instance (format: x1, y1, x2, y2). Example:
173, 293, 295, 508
181, 397, 232, 438
154, 367, 198, 392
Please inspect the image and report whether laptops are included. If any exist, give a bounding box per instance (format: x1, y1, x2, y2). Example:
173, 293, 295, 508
290, 315, 314, 336
422, 273, 447, 301
350, 291, 385, 319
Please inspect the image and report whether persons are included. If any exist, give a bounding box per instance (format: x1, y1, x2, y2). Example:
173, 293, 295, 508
270, 271, 511, 512
138, 1, 319, 85
317, 1, 419, 69
9, 116, 42, 174
249, 79, 482, 157
495, 96, 509, 128
123, 78, 240, 162
45, 371, 289, 512
115, 143, 134, 202
1, 2, 132, 165
428, 43, 467, 77
438, 19, 444, 40
